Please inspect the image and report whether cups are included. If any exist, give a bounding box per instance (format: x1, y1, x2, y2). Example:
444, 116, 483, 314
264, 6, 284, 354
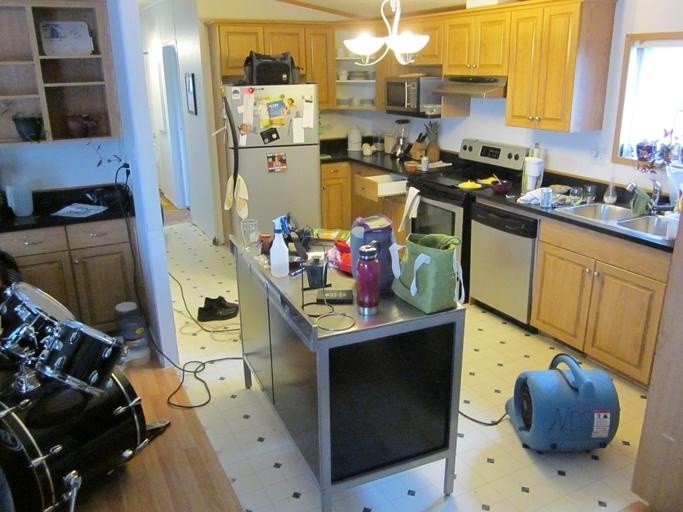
5, 183, 34, 217
60, 115, 95, 137
569, 187, 583, 206
337, 71, 347, 81
420, 156, 428, 171
583, 184, 596, 203
538, 188, 553, 208
240, 218, 261, 255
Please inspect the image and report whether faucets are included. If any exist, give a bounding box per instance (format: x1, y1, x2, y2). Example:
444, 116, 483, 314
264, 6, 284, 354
626, 176, 662, 214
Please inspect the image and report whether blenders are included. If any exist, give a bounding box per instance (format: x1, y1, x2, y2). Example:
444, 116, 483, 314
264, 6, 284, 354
390, 119, 411, 157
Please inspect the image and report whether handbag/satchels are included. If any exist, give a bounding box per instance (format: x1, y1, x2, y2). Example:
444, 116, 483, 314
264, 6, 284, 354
350, 215, 395, 290
391, 234, 461, 314
243, 50, 291, 84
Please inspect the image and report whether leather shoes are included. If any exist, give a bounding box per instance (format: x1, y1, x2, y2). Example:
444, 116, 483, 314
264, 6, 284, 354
198, 296, 239, 321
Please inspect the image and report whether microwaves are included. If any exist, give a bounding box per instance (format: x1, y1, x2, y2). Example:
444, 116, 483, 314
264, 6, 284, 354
381, 72, 441, 118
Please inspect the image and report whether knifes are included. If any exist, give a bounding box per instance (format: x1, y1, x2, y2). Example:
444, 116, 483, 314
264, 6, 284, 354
414, 131, 428, 143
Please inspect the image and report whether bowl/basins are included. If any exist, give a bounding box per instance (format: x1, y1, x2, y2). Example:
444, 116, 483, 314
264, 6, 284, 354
335, 96, 352, 106
490, 180, 512, 195
348, 70, 368, 80
423, 104, 440, 116
361, 99, 374, 106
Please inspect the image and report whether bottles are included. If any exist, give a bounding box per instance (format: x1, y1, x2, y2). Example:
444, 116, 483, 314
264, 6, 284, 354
373, 130, 383, 152
353, 239, 382, 316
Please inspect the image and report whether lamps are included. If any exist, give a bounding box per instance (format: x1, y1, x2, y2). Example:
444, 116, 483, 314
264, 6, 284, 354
343, 0, 431, 66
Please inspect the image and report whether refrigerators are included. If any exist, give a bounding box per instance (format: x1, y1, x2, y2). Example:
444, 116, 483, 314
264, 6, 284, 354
223, 82, 322, 247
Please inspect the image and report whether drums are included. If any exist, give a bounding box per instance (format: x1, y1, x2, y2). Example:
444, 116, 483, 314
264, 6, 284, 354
0, 365, 147, 511
0, 276, 75, 361
36, 318, 127, 397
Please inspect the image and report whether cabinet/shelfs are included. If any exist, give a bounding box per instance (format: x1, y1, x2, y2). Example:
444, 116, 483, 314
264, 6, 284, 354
0, 217, 141, 334
334, 22, 381, 111
381, 12, 470, 118
442, 4, 511, 77
219, 19, 306, 76
530, 216, 672, 387
305, 22, 336, 111
505, 0, 617, 133
321, 161, 352, 230
0, 0, 123, 146
351, 162, 410, 261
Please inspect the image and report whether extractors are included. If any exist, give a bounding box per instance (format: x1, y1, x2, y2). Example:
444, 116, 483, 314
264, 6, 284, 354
431, 76, 506, 99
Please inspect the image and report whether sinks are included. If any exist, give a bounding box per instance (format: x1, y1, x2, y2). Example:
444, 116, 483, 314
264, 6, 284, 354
555, 203, 644, 223
616, 212, 681, 248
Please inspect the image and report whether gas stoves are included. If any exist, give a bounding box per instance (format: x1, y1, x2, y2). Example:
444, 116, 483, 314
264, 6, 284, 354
409, 167, 516, 197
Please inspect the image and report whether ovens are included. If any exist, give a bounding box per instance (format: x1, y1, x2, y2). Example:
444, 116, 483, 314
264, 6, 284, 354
404, 183, 470, 304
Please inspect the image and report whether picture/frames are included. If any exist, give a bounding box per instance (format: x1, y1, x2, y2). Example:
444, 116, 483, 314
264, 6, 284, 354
184, 73, 198, 116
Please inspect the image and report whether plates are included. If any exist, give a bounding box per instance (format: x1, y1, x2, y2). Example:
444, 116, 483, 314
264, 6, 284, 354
454, 183, 486, 193
475, 178, 492, 187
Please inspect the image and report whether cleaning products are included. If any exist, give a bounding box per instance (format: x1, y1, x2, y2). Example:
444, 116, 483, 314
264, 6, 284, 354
270, 216, 289, 277
603, 175, 617, 204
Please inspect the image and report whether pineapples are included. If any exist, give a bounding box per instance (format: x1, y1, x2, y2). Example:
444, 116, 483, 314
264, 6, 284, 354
424, 120, 440, 162
